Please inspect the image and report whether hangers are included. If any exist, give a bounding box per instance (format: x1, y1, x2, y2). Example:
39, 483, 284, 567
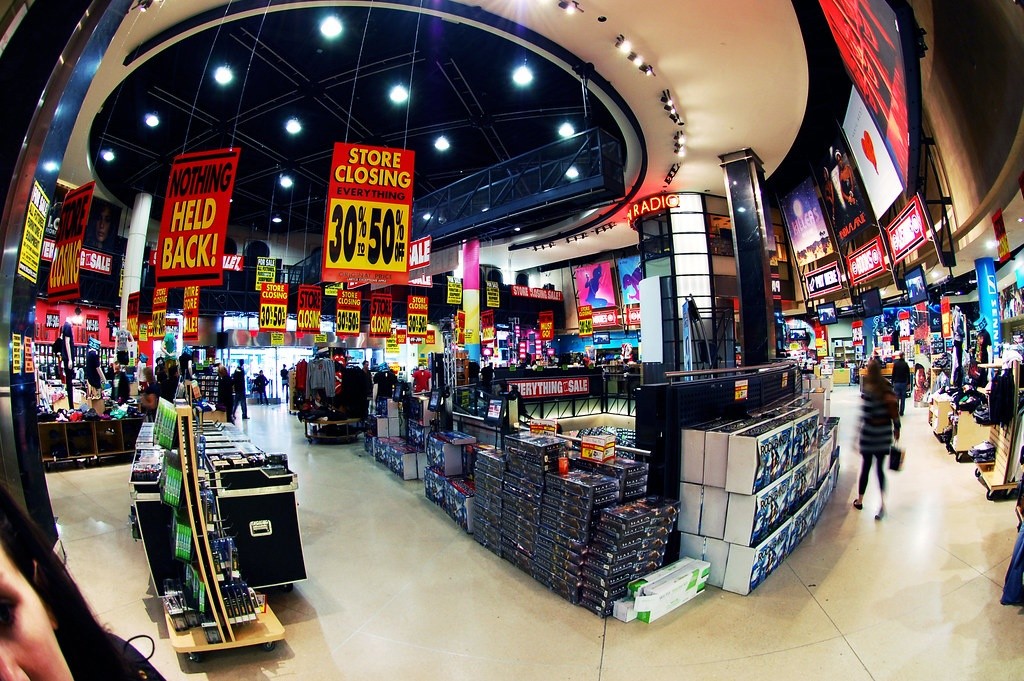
990, 367, 1015, 386
285, 353, 399, 379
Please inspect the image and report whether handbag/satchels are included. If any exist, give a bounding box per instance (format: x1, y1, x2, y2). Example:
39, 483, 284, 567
885, 380, 899, 419
889, 439, 904, 470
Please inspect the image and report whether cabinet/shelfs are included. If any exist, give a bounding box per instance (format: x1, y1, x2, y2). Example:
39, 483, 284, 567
128, 422, 308, 606
950, 403, 991, 454
927, 395, 955, 436
303, 417, 362, 444
190, 374, 223, 407
36, 412, 146, 469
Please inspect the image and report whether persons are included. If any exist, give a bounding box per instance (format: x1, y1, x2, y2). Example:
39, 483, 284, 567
61, 317, 75, 413
107, 360, 130, 405
372, 361, 397, 399
913, 363, 927, 402
85, 347, 109, 398
280, 363, 289, 407
161, 328, 178, 382
852, 355, 906, 521
232, 359, 250, 419
218, 365, 235, 425
116, 326, 134, 372
481, 362, 495, 398
362, 360, 372, 377
178, 351, 196, 380
798, 333, 810, 348
412, 365, 432, 392
891, 351, 911, 417
254, 370, 271, 406
137, 353, 150, 394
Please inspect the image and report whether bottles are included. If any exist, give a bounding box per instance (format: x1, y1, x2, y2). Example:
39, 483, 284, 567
558, 443, 568, 476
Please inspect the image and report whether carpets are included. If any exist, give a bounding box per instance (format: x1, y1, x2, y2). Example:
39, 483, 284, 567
247, 397, 282, 404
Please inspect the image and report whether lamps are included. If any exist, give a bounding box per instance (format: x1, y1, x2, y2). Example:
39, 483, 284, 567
673, 130, 685, 158
559, 1, 577, 16
71, 305, 84, 324
614, 33, 657, 77
660, 89, 684, 128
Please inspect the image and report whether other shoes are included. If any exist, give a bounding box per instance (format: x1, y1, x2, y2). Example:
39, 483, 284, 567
876, 511, 883, 519
854, 500, 862, 510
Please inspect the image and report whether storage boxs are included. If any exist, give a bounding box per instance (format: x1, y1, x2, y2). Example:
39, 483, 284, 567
801, 378, 832, 390
363, 393, 840, 624
